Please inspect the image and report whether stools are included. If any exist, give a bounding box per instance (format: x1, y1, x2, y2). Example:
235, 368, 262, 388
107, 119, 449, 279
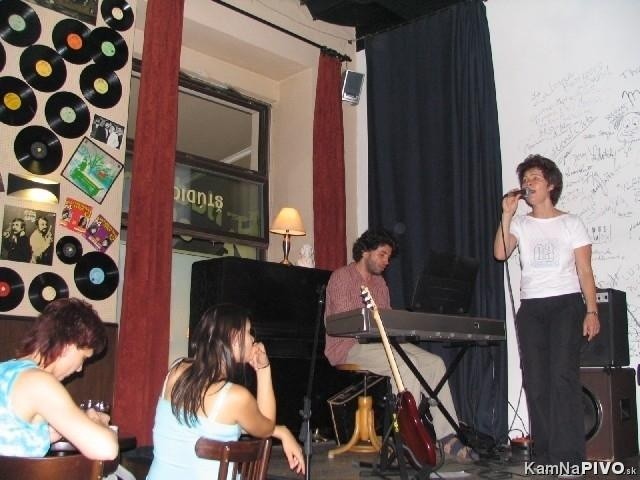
328, 364, 394, 460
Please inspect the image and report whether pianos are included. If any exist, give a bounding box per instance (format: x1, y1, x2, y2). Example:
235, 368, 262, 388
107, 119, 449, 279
326, 308, 506, 341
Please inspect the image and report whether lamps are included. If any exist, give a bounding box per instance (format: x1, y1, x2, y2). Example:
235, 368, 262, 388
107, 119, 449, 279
270, 207, 306, 265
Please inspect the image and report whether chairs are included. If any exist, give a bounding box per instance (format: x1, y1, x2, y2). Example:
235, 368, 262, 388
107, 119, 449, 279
2, 455, 106, 479
195, 437, 271, 479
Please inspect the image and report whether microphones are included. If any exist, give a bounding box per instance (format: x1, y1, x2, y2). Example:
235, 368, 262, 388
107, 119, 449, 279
507, 188, 533, 199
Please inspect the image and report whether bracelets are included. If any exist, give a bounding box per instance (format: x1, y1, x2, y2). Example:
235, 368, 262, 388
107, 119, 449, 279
259, 362, 270, 369
587, 310, 599, 315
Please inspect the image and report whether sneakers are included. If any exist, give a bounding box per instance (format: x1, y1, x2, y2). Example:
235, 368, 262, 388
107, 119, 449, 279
558, 471, 584, 478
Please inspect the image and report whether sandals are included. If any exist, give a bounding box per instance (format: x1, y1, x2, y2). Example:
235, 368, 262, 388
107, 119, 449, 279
437, 434, 478, 464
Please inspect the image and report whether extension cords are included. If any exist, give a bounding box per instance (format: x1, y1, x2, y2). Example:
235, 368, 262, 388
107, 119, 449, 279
507, 435, 529, 449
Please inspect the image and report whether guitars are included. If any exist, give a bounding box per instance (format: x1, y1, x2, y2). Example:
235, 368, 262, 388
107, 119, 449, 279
360, 285, 437, 475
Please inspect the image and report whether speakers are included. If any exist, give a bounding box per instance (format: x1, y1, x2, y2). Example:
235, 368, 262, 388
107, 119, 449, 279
339, 70, 364, 106
579, 368, 640, 463
579, 287, 631, 369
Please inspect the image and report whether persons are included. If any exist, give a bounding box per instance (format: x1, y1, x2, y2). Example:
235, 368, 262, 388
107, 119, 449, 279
90, 117, 123, 149
0, 298, 119, 461
145, 305, 305, 480
323, 230, 479, 462
3, 218, 32, 263
29, 216, 53, 263
494, 156, 600, 479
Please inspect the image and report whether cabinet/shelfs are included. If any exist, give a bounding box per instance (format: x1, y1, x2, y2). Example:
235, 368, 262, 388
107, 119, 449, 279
189, 255, 363, 444
